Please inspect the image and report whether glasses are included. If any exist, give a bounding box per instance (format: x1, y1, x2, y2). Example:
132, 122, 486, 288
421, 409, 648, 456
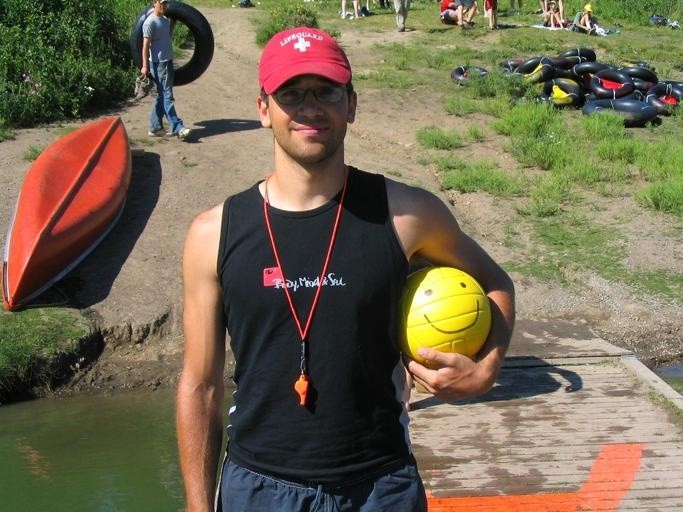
275, 86, 349, 105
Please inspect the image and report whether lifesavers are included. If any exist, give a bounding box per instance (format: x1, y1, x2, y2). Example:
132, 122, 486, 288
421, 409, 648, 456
132, 1, 213, 87
450, 49, 683, 126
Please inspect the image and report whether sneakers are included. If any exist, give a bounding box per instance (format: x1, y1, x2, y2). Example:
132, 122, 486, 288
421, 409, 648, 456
134, 75, 152, 99
177, 127, 191, 139
586, 27, 596, 34
148, 130, 171, 137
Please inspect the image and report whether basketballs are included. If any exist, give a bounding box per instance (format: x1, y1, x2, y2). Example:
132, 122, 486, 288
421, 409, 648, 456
399, 266, 491, 370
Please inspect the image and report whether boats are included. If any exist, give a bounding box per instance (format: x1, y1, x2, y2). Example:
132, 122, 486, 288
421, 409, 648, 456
2, 115, 132, 312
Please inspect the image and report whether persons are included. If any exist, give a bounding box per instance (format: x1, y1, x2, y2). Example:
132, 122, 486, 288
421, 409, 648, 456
141, 0, 191, 140
439, 0, 497, 30
539, 0, 596, 35
394, 0, 410, 32
174, 27, 513, 511
339, 0, 390, 19
510, 0, 522, 16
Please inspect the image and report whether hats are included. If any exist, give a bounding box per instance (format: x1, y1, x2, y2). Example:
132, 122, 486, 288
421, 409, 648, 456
259, 27, 352, 95
585, 4, 592, 11
